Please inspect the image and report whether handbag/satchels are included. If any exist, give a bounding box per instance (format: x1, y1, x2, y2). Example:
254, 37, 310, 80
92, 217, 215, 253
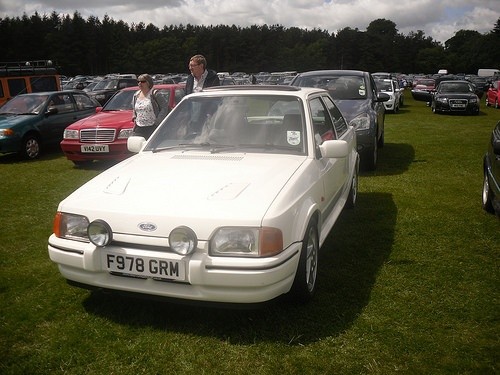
151, 88, 172, 119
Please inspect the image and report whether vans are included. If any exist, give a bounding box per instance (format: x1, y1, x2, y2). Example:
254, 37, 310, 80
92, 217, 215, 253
0, 60, 60, 108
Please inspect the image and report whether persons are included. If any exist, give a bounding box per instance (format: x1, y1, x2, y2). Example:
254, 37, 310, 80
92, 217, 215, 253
131, 73, 169, 140
185, 54, 220, 132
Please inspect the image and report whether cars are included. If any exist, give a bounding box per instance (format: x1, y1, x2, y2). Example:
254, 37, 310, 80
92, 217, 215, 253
428, 80, 481, 116
47, 84, 361, 305
359, 79, 405, 113
60, 83, 189, 167
61, 71, 298, 108
370, 72, 500, 109
482, 121, 500, 216
263, 69, 390, 170
0, 90, 103, 160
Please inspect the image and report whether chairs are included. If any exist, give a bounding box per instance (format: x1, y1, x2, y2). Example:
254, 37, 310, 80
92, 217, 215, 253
199, 112, 336, 153
306, 81, 363, 100
26, 98, 67, 114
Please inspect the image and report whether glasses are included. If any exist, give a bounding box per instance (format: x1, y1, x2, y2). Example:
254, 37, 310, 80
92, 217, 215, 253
136, 80, 147, 84
